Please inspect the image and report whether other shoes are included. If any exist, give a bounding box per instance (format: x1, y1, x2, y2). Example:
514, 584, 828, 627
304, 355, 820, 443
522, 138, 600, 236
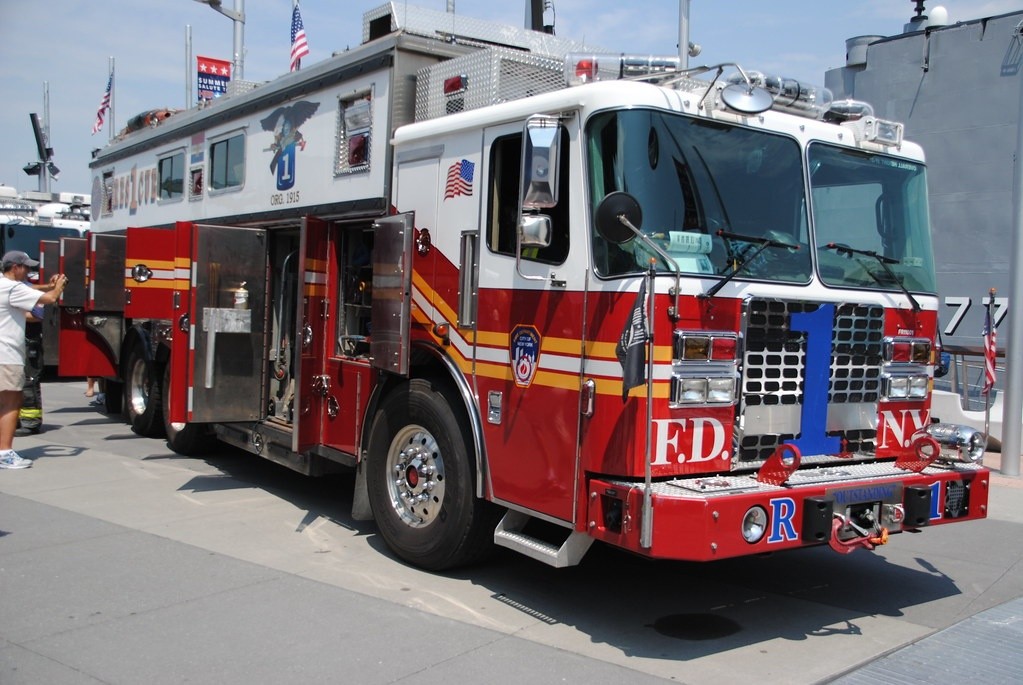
86, 389, 94, 396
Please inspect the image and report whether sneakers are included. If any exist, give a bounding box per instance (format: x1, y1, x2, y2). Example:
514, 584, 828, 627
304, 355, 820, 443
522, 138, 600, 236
0, 449, 33, 469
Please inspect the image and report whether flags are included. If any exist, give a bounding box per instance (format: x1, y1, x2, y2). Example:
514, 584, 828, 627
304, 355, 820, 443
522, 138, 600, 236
615, 273, 646, 403
982, 303, 996, 393
290, 5, 309, 70
92, 74, 112, 135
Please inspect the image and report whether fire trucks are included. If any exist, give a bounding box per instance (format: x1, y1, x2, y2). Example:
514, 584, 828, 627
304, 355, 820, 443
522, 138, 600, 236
37, 0, 997, 571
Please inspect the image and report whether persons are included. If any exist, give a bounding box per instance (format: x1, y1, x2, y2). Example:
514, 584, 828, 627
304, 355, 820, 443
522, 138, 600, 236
0, 251, 69, 470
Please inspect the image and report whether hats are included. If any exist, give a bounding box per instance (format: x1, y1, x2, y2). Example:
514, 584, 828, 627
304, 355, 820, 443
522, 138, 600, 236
1, 250, 40, 269
